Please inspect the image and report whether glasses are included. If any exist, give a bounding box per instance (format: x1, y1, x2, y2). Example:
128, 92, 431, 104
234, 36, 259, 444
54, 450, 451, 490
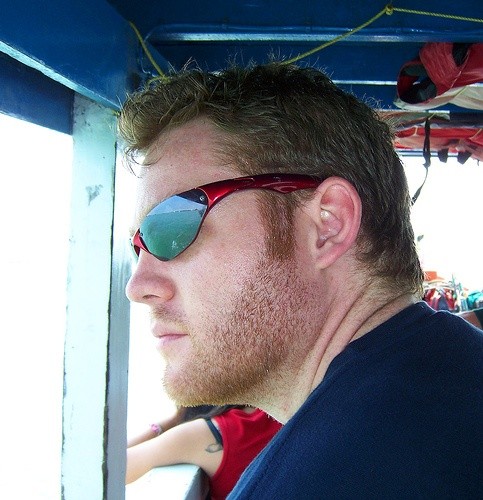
129, 173, 323, 262
